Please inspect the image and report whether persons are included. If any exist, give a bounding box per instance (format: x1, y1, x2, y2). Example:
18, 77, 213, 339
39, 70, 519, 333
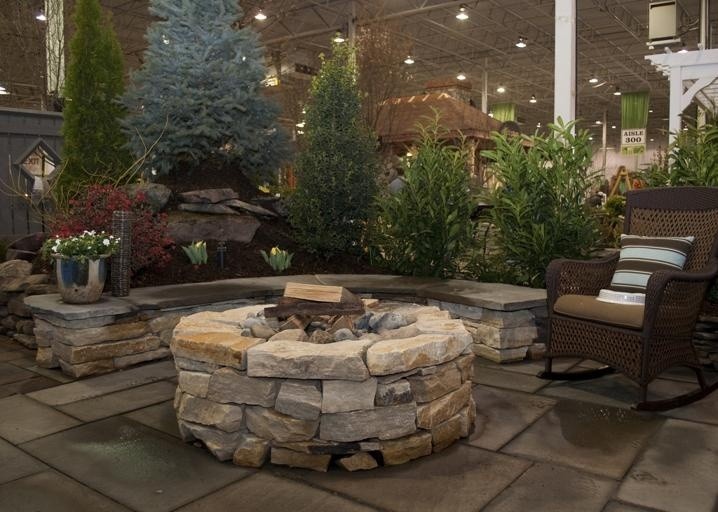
385, 165, 408, 199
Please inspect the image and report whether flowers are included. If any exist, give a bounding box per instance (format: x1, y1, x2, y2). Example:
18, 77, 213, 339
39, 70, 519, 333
34, 225, 123, 264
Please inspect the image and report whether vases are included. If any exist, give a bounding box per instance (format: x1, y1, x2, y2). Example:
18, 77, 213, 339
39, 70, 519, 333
54, 254, 109, 303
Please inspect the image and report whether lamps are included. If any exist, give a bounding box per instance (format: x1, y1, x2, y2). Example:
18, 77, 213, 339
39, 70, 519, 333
35, 7, 46, 23
254, 8, 269, 23
454, 5, 470, 20
331, 31, 345, 45
528, 94, 540, 104
514, 36, 528, 49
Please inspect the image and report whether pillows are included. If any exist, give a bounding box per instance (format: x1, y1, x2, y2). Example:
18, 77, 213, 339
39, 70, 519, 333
607, 228, 695, 298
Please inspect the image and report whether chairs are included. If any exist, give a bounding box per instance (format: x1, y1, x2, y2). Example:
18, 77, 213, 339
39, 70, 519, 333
533, 185, 718, 415
